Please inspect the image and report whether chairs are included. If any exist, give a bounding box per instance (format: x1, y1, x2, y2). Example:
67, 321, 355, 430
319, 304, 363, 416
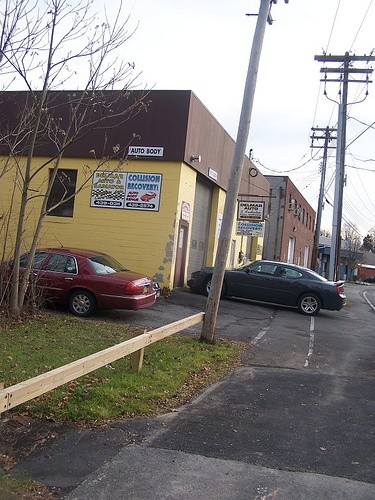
280, 268, 288, 278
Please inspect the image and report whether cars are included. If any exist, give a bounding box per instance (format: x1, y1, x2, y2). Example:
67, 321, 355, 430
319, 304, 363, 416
1, 246, 158, 320
140, 191, 156, 202
187, 258, 346, 317
